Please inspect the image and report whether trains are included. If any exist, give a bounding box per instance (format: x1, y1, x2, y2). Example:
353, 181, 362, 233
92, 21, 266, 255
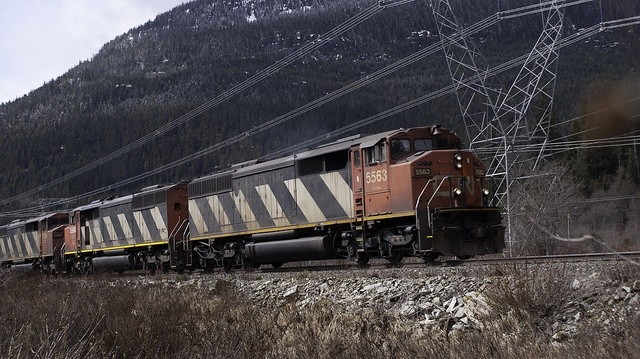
0, 124, 506, 274
